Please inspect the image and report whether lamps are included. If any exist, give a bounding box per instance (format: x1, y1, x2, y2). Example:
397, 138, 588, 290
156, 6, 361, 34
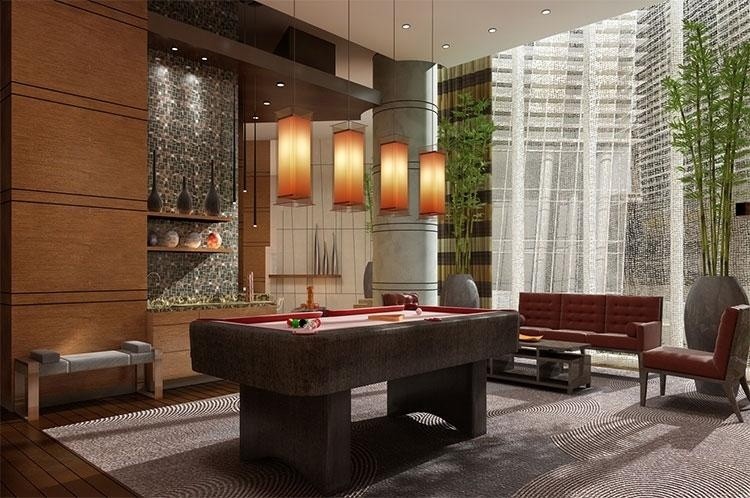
271, 1, 450, 216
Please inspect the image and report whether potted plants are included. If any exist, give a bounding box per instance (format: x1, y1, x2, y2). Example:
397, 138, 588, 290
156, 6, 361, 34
363, 155, 373, 298
430, 94, 499, 310
659, 17, 750, 396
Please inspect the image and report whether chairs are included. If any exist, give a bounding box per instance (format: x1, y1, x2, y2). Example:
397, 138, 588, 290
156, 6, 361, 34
637, 305, 749, 422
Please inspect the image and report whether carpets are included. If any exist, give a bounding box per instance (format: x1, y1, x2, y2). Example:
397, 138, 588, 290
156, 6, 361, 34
41, 349, 748, 497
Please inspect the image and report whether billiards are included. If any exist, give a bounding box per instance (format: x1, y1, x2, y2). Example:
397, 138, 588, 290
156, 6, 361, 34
416, 308, 422, 315
286, 316, 321, 332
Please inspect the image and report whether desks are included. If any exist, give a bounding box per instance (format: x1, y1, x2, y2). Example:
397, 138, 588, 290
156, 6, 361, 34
188, 305, 523, 495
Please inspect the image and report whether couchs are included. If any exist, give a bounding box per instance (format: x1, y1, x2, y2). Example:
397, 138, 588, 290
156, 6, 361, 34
513, 292, 664, 359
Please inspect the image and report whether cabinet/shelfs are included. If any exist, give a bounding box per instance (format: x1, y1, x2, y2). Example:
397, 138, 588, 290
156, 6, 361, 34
147, 211, 232, 253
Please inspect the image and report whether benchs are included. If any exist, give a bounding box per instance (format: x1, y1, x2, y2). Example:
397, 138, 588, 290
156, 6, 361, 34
14, 339, 164, 422
